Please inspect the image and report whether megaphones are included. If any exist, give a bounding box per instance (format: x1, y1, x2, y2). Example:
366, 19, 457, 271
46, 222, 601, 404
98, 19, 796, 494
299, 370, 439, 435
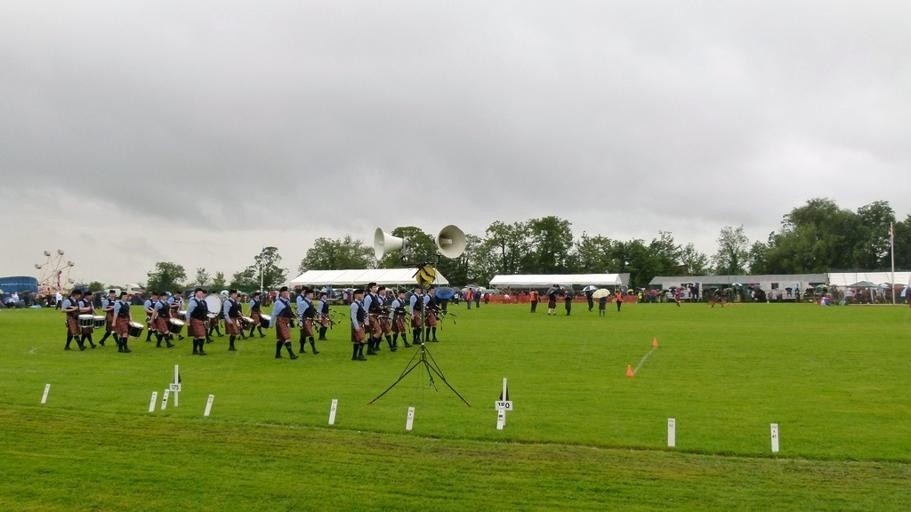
433, 225, 466, 258
374, 226, 410, 261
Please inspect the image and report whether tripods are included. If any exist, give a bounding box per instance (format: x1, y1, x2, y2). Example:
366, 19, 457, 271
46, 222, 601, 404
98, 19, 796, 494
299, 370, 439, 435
367, 253, 472, 408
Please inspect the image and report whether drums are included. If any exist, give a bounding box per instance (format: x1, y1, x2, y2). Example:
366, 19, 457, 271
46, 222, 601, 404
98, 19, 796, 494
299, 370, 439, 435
177, 311, 188, 320
206, 313, 219, 327
168, 318, 184, 333
128, 322, 144, 337
240, 316, 254, 328
259, 314, 271, 328
205, 293, 228, 319
78, 313, 106, 329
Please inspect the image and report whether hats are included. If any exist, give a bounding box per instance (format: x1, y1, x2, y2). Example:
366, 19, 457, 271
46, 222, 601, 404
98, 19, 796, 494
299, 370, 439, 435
83, 291, 92, 296
255, 293, 260, 296
160, 291, 168, 296
426, 285, 434, 291
376, 285, 385, 293
121, 292, 127, 295
175, 289, 182, 294
237, 294, 240, 297
411, 285, 422, 293
353, 290, 365, 294
151, 290, 158, 295
229, 289, 236, 294
110, 290, 115, 293
398, 287, 408, 293
305, 289, 314, 293
72, 289, 82, 294
319, 293, 328, 296
302, 287, 309, 291
366, 283, 376, 292
194, 288, 208, 294
279, 287, 287, 293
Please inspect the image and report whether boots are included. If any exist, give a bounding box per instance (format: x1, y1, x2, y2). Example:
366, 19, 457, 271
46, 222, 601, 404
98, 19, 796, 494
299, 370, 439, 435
319, 327, 327, 340
146, 329, 184, 348
204, 324, 224, 344
240, 329, 248, 339
112, 333, 132, 352
309, 336, 319, 354
275, 341, 283, 358
229, 335, 238, 351
64, 334, 96, 351
192, 339, 207, 355
99, 332, 111, 346
249, 327, 255, 337
351, 327, 440, 360
299, 342, 307, 353
285, 342, 299, 359
257, 325, 266, 337
